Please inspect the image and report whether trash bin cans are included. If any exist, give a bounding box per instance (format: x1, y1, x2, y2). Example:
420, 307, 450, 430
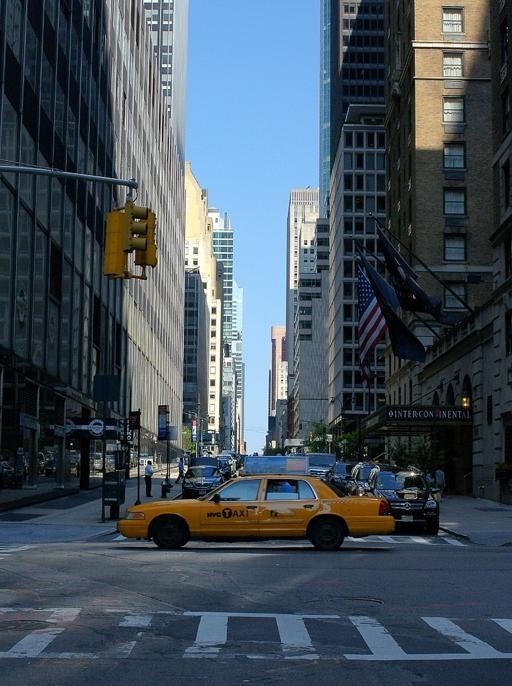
103, 470, 126, 519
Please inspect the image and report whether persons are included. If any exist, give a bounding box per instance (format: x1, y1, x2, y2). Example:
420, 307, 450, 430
145, 461, 156, 498
494, 462, 506, 504
435, 467, 446, 498
175, 457, 185, 485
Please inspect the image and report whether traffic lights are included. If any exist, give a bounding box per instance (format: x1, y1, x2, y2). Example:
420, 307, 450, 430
125, 202, 147, 250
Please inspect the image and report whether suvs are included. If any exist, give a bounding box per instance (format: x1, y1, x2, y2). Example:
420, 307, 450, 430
365, 465, 442, 536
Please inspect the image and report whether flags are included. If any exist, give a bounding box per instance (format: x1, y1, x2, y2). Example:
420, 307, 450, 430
353, 265, 388, 370
377, 227, 460, 329
358, 254, 427, 364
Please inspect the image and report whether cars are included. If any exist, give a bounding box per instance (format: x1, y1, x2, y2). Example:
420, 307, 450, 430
307, 451, 337, 480
345, 462, 397, 497
181, 453, 254, 498
326, 462, 360, 492
116, 474, 396, 553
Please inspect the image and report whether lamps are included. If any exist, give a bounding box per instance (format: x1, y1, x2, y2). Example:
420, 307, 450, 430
462, 390, 470, 409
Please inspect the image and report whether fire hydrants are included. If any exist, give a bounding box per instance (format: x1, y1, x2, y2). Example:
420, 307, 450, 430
161, 481, 170, 498
